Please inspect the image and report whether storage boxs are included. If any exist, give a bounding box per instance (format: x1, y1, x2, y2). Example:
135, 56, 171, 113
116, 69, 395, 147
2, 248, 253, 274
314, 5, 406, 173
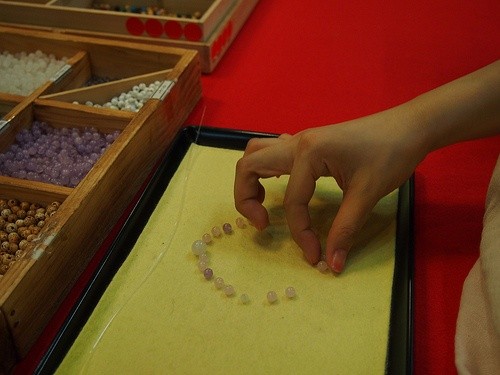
0, 0, 260, 375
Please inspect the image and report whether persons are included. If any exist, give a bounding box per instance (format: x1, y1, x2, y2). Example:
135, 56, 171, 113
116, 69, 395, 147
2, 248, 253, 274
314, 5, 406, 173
234, 58, 500, 273
